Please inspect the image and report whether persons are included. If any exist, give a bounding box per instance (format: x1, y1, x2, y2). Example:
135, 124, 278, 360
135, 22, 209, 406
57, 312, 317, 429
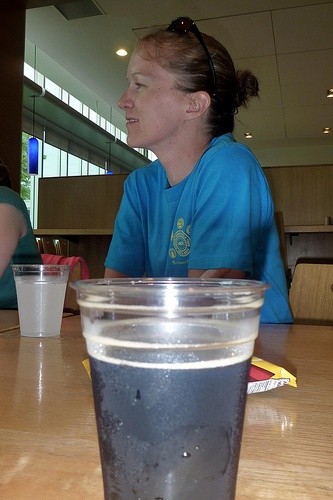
0, 160, 45, 310
104, 17, 294, 325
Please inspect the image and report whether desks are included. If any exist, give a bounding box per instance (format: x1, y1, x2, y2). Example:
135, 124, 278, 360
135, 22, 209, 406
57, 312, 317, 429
0, 310, 332, 500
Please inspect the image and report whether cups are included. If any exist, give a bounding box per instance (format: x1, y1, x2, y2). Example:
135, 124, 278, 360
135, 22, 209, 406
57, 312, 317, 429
15, 337, 63, 408
70, 279, 268, 500
11, 264, 71, 338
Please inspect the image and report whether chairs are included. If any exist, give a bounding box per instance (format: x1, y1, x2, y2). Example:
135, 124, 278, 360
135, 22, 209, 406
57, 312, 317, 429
41, 253, 81, 315
287, 263, 333, 326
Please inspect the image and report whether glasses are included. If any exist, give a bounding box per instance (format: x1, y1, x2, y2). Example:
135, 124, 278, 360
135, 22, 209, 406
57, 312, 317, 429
167, 16, 217, 98
